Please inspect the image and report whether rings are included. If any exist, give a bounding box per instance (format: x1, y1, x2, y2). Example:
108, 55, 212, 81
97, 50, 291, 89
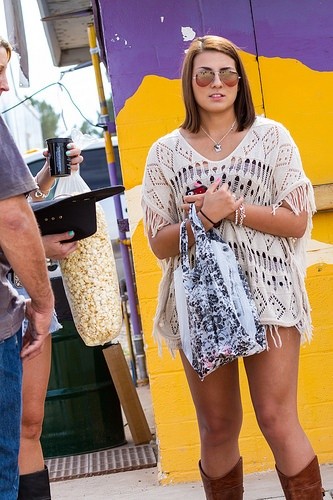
233, 193, 238, 200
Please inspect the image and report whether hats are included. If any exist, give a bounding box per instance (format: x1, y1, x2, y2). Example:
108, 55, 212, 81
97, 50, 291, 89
26, 185, 125, 244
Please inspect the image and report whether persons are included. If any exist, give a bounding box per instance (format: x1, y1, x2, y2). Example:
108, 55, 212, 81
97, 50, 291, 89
0, 141, 84, 500
140, 35, 325, 500
0, 42, 55, 500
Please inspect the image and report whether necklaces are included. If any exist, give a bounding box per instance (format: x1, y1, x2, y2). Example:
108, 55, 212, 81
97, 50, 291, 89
200, 117, 237, 152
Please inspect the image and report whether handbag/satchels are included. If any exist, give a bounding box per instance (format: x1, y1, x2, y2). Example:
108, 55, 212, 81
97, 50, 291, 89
173, 202, 268, 382
101, 339, 154, 446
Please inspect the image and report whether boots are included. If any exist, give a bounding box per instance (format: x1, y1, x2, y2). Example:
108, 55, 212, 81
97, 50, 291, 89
198, 456, 244, 500
17, 464, 52, 500
274, 454, 326, 500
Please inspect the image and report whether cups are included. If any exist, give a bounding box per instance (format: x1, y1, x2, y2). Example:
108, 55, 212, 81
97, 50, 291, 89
47, 138, 74, 178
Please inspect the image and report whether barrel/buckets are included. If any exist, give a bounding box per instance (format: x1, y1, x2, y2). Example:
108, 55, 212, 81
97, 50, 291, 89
40, 320, 128, 460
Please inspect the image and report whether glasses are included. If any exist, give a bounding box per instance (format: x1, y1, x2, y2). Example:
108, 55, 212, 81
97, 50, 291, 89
192, 70, 242, 87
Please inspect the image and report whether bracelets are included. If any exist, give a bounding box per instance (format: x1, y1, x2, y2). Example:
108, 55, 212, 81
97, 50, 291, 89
239, 203, 247, 228
33, 174, 50, 199
235, 209, 239, 226
199, 209, 215, 225
